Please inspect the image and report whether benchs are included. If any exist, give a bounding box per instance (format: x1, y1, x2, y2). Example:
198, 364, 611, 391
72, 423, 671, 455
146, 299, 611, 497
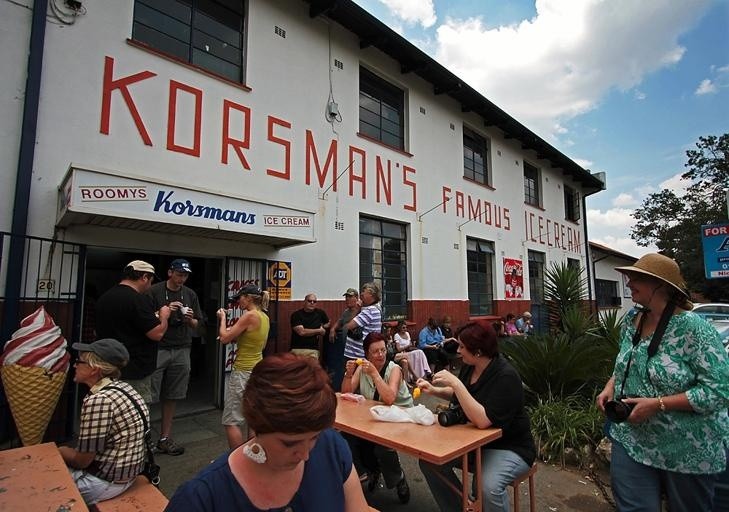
94, 474, 170, 512
460, 462, 539, 511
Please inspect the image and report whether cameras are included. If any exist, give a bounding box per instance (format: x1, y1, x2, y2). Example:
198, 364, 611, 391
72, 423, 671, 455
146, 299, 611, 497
606, 392, 640, 424
437, 407, 468, 428
436, 341, 445, 348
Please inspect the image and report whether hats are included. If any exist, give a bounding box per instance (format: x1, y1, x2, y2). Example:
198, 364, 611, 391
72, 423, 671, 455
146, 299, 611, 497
123, 260, 163, 282
170, 259, 192, 273
614, 253, 689, 304
72, 339, 129, 368
231, 284, 262, 299
343, 288, 357, 296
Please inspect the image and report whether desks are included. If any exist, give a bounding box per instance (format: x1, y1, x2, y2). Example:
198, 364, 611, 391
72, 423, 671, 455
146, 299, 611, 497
333, 398, 503, 512
0, 441, 92, 511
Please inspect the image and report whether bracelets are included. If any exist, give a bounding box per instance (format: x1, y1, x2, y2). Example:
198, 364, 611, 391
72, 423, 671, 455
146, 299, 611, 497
659, 396, 666, 411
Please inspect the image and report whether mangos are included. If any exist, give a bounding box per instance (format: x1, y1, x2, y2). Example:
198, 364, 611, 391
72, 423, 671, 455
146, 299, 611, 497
355, 358, 363, 366
413, 388, 421, 400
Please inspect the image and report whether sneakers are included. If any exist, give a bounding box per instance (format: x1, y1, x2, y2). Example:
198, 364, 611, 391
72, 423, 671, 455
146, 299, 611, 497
157, 437, 184, 456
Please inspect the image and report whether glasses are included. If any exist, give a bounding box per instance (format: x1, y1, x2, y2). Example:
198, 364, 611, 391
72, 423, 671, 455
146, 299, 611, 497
75, 358, 89, 366
307, 300, 317, 303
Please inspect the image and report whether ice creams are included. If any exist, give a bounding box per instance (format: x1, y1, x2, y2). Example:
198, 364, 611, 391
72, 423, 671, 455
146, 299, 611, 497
1, 306, 71, 448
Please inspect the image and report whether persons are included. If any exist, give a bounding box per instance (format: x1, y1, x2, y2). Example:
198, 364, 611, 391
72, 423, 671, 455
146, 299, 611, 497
415, 320, 537, 512
216, 286, 270, 450
419, 315, 460, 375
595, 251, 729, 511
506, 269, 524, 299
56, 257, 206, 507
492, 311, 532, 337
163, 350, 370, 511
289, 282, 432, 505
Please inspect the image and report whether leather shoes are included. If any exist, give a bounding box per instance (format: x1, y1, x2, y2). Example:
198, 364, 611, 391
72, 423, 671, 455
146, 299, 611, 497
397, 469, 410, 503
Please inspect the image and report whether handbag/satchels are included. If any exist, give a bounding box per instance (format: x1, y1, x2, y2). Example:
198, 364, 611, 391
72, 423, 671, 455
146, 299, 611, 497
348, 327, 363, 341
143, 461, 161, 488
